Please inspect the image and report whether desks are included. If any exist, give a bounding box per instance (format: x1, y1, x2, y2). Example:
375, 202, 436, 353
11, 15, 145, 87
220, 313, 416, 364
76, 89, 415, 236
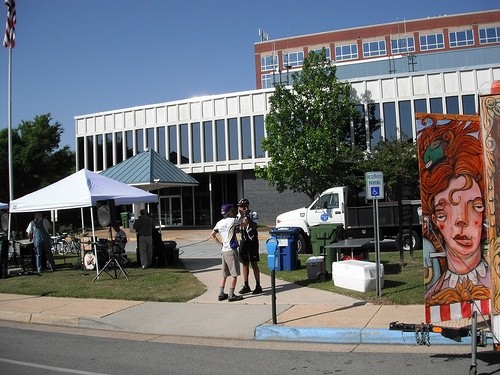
324, 238, 374, 261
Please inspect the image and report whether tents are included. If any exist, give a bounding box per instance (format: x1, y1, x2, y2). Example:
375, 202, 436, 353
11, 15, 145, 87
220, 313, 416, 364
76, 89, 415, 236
8, 168, 158, 279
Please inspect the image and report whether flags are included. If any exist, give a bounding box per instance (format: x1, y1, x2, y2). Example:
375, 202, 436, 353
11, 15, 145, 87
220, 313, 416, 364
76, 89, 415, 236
3, 0, 17, 48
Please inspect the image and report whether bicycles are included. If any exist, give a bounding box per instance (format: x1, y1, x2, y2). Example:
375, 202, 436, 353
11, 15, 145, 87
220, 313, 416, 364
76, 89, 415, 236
51, 231, 81, 255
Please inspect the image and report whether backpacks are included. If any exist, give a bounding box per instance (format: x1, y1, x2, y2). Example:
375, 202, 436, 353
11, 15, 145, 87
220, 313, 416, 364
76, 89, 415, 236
33, 219, 50, 247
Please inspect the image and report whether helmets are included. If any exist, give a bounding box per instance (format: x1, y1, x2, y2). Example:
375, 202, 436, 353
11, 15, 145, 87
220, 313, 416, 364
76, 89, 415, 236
237, 198, 249, 207
220, 204, 233, 214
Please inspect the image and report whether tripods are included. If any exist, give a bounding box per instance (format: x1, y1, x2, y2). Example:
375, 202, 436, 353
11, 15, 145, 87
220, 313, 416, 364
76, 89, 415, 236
92, 225, 130, 282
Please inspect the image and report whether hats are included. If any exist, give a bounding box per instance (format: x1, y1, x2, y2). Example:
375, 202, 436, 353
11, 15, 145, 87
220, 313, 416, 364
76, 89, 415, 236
140, 209, 147, 214
35, 213, 42, 218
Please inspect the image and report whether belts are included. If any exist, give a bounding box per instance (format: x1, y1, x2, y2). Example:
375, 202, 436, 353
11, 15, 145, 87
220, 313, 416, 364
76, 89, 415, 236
139, 234, 151, 237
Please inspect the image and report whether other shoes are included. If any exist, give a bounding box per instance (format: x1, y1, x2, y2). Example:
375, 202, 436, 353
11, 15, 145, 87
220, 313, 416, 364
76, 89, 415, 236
228, 294, 243, 302
142, 266, 146, 269
252, 286, 262, 294
238, 286, 250, 294
218, 293, 228, 300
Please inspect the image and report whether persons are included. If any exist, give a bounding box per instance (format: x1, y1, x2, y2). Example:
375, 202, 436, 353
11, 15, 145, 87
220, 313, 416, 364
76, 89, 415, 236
133, 209, 152, 268
113, 224, 127, 246
210, 204, 248, 302
26, 211, 56, 273
235, 198, 262, 294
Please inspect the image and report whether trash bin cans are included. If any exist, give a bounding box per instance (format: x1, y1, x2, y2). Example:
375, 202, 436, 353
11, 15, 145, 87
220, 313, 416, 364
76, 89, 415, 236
308, 224, 344, 274
270, 226, 301, 271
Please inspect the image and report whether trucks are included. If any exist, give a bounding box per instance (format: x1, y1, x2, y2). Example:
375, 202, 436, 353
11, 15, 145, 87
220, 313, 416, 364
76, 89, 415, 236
275, 184, 422, 255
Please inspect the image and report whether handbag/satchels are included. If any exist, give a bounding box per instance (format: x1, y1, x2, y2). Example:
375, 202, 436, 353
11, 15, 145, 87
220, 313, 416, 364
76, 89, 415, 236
230, 231, 239, 249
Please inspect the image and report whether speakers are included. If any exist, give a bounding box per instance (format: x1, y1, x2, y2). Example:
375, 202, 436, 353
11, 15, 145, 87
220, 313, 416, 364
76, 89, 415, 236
96, 199, 117, 227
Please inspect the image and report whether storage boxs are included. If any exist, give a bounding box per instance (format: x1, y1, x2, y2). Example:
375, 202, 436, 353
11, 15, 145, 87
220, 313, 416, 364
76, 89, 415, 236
332, 259, 385, 292
306, 255, 327, 281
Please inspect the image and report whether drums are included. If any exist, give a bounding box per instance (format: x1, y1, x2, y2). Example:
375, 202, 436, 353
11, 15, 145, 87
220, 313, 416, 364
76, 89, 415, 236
83, 250, 107, 270
